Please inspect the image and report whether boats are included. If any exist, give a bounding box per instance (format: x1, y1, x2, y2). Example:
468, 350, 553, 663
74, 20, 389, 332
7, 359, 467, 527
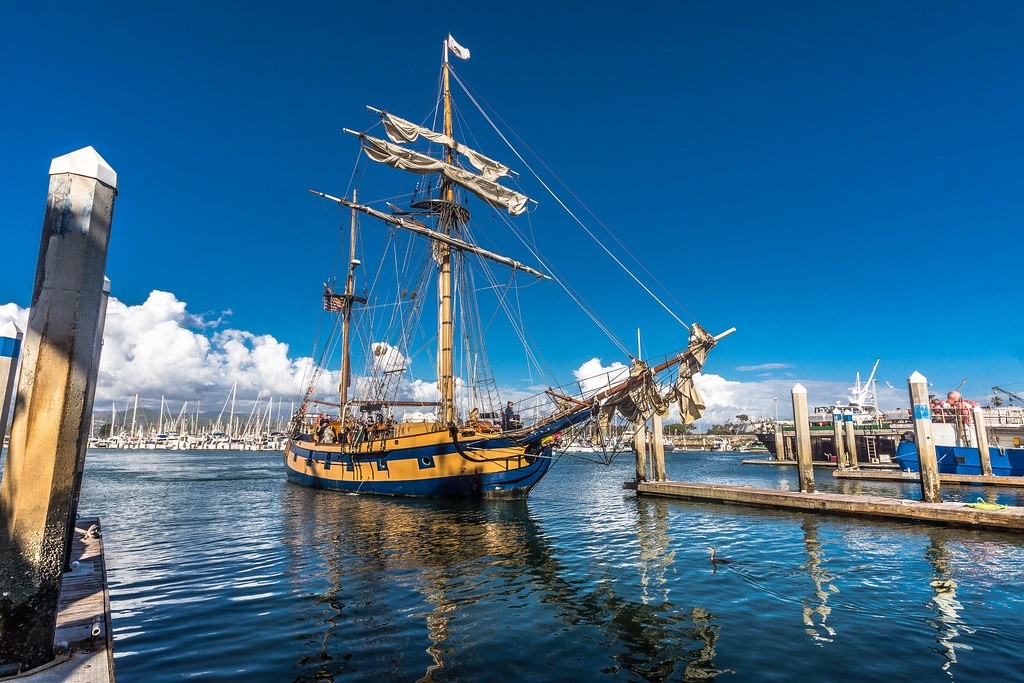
709, 359, 1024, 477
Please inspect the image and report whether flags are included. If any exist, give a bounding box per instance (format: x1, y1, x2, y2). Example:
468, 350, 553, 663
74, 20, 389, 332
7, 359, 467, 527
447, 33, 470, 61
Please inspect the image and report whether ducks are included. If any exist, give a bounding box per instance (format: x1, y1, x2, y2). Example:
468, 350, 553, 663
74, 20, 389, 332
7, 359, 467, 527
706, 547, 731, 564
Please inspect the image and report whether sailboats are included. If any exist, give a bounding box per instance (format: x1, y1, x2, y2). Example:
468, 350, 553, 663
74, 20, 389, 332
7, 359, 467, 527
86, 381, 673, 452
284, 33, 737, 499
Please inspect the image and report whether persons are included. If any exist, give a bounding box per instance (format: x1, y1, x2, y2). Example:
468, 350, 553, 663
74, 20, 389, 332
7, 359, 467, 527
469, 407, 480, 428
315, 411, 397, 444
505, 401, 514, 431
